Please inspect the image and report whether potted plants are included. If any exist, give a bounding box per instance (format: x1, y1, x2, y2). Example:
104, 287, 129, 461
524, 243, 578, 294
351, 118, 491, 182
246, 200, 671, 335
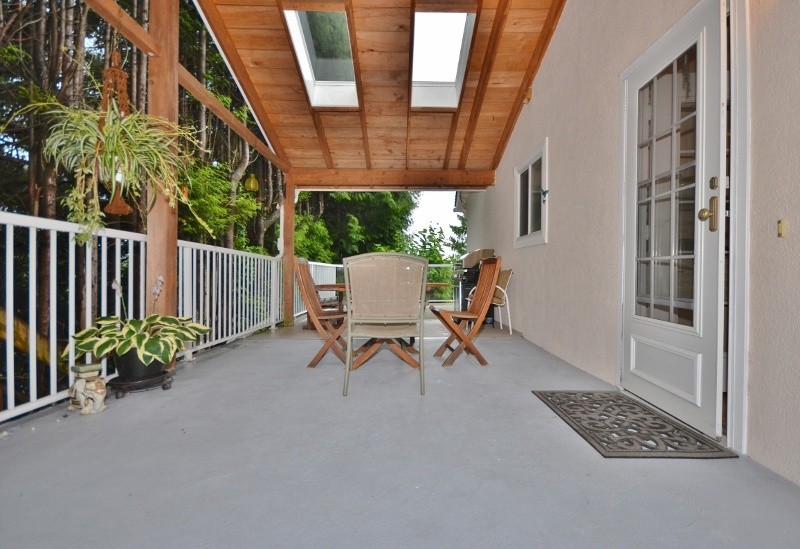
107, 349, 173, 400
3, 82, 215, 248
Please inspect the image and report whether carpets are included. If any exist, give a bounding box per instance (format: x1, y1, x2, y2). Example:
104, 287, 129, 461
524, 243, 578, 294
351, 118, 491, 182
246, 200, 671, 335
532, 390, 739, 458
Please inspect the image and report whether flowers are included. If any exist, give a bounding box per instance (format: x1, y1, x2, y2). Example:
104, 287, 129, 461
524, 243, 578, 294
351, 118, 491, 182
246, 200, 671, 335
59, 275, 211, 365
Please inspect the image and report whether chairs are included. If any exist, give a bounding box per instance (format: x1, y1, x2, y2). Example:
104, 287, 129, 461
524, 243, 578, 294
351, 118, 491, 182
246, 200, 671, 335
342, 252, 429, 396
464, 270, 513, 336
294, 255, 359, 368
306, 275, 345, 330
430, 256, 502, 367
